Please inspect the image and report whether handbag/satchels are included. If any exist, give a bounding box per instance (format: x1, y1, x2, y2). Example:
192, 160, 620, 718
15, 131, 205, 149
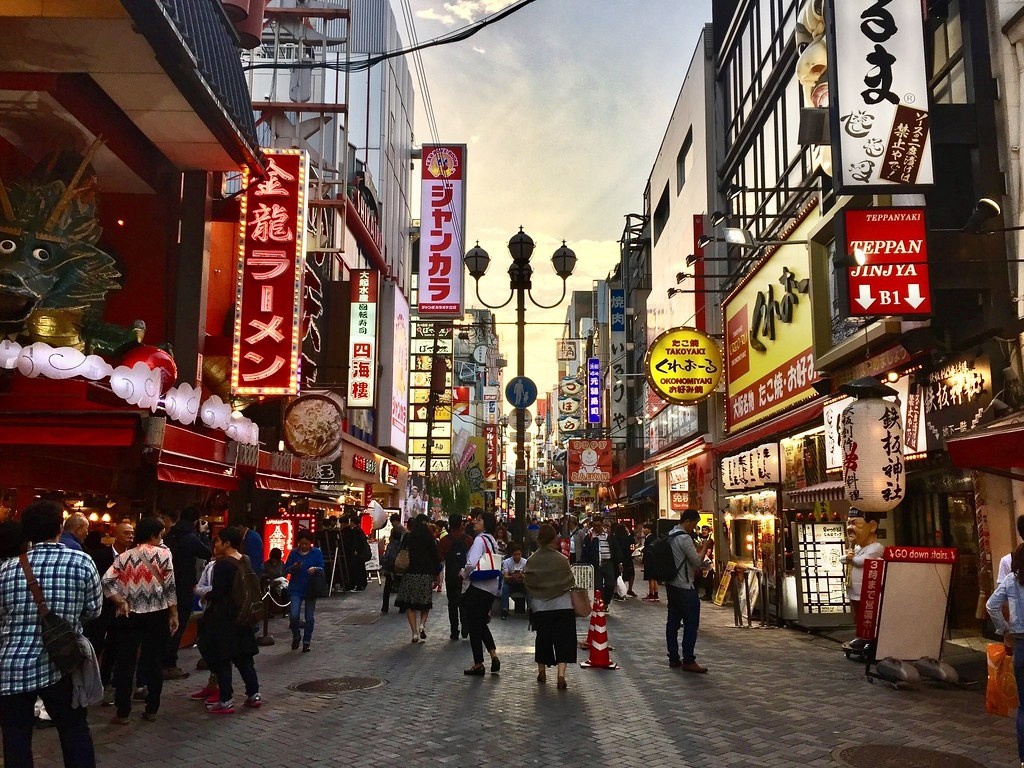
395, 533, 410, 570
39, 611, 105, 708
570, 584, 592, 617
985, 642, 1020, 721
614, 571, 627, 597
468, 535, 502, 581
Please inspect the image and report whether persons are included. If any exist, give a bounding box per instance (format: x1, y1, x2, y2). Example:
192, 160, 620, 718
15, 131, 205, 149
458, 511, 500, 675
406, 488, 423, 517
438, 514, 474, 640
0, 520, 30, 559
83, 517, 180, 726
58, 512, 89, 551
666, 509, 712, 674
382, 510, 714, 642
235, 521, 324, 652
986, 542, 1024, 768
996, 514, 1024, 584
323, 515, 367, 593
523, 524, 577, 690
839, 506, 885, 650
190, 526, 262, 715
162, 506, 211, 680
0, 499, 103, 768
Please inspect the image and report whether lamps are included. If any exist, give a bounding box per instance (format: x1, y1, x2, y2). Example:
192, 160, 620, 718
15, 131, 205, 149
614, 372, 647, 385
667, 182, 822, 296
833, 247, 1024, 268
636, 401, 669, 424
981, 387, 1010, 415
963, 197, 1024, 236
797, 105, 831, 146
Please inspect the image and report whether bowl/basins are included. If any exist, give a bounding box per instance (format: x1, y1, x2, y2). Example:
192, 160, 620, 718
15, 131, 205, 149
283, 394, 343, 462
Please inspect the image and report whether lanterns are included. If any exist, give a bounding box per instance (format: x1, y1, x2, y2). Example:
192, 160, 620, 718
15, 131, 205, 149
727, 490, 776, 518
840, 397, 906, 513
721, 443, 786, 489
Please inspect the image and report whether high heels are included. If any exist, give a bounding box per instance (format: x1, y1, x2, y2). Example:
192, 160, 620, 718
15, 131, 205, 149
491, 656, 500, 673
464, 664, 486, 676
411, 632, 419, 642
419, 624, 426, 639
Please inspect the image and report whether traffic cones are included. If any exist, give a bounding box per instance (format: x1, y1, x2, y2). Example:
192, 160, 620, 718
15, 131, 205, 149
580, 600, 619, 670
580, 590, 615, 651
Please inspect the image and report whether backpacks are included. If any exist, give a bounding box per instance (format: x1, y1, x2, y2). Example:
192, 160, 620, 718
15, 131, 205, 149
643, 531, 694, 582
222, 555, 265, 627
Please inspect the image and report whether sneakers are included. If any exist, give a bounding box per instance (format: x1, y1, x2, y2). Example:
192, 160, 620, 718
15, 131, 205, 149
190, 683, 263, 714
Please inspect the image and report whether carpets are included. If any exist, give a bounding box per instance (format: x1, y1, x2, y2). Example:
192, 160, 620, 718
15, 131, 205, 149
946, 636, 1004, 653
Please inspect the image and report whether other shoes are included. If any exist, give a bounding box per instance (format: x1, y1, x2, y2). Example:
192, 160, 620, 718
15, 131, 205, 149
669, 657, 708, 673
450, 630, 460, 640
141, 710, 157, 721
501, 609, 509, 619
537, 671, 546, 682
110, 714, 131, 724
104, 658, 210, 704
461, 621, 468, 638
291, 635, 302, 649
557, 676, 567, 689
432, 583, 441, 592
303, 642, 311, 652
381, 604, 406, 613
613, 590, 712, 602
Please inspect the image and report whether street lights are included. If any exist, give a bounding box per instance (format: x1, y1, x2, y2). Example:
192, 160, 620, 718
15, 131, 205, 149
462, 225, 578, 613
513, 444, 531, 523
